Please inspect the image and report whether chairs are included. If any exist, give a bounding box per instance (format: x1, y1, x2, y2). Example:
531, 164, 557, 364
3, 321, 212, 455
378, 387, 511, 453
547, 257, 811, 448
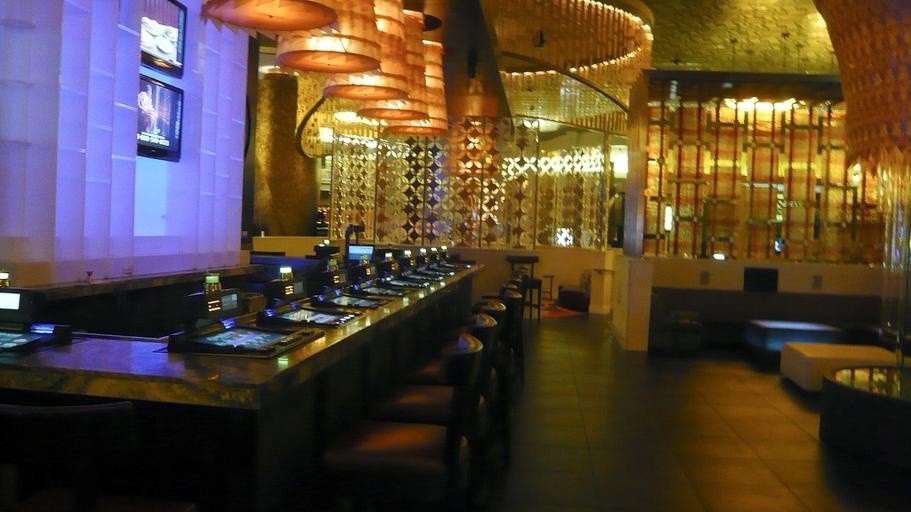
652, 291, 705, 353
0, 398, 198, 511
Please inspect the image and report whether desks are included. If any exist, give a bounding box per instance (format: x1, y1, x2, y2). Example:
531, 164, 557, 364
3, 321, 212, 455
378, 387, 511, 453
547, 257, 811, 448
743, 319, 909, 472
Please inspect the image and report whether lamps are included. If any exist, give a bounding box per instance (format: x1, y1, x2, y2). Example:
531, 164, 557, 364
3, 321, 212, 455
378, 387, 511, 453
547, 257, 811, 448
200, 1, 448, 135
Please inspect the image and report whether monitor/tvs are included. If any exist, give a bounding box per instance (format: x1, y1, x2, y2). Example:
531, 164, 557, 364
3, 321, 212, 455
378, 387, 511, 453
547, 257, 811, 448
311, 289, 382, 309
168, 316, 324, 359
137, 73, 184, 162
256, 301, 363, 329
347, 244, 471, 297
0, 325, 73, 354
140, 0, 187, 79
